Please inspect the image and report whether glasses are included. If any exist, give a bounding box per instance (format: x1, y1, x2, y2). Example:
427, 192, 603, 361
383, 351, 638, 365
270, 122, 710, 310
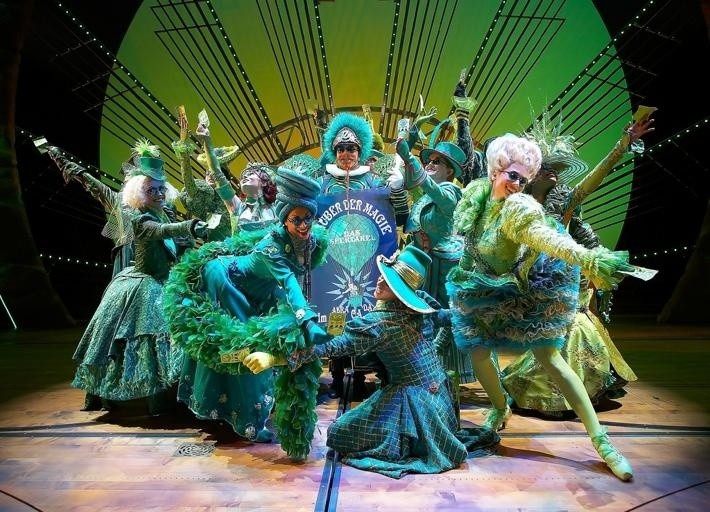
285, 214, 314, 225
501, 169, 529, 187
424, 157, 449, 168
336, 142, 358, 155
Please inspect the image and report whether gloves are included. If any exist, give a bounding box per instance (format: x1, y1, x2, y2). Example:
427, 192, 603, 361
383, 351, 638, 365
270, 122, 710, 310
243, 351, 286, 374
609, 260, 634, 283
47, 145, 88, 186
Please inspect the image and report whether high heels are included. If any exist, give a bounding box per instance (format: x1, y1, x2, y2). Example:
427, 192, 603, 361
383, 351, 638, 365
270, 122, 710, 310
590, 430, 633, 484
482, 402, 512, 435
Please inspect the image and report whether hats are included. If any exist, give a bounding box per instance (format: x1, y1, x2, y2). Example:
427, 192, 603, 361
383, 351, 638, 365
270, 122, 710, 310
128, 154, 167, 182
330, 125, 364, 154
419, 141, 467, 179
537, 150, 590, 186
197, 145, 240, 171
274, 166, 319, 225
376, 243, 442, 315
